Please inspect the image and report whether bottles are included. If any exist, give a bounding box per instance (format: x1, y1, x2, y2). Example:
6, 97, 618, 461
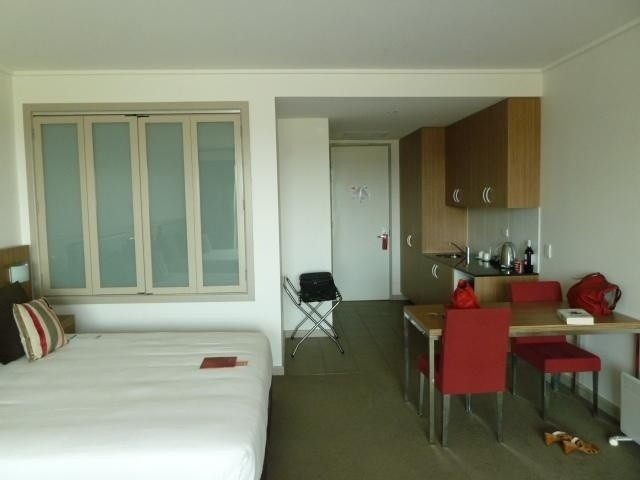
524, 239, 535, 273
513, 258, 523, 274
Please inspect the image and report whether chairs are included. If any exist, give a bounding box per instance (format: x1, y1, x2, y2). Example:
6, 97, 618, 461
283, 276, 345, 357
508, 282, 601, 420
418, 308, 510, 447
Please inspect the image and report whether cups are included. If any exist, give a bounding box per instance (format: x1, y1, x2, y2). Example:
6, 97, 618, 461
479, 251, 485, 258
483, 253, 491, 259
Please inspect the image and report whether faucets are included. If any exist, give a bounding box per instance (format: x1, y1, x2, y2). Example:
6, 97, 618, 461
454, 255, 470, 267
448, 240, 470, 255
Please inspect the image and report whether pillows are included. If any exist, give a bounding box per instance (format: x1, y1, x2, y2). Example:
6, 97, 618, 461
0, 281, 33, 365
13, 298, 68, 362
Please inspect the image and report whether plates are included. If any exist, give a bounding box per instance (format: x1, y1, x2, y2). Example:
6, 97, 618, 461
481, 258, 492, 262
474, 257, 484, 260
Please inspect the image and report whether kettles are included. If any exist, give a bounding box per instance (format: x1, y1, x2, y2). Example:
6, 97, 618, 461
500, 241, 517, 269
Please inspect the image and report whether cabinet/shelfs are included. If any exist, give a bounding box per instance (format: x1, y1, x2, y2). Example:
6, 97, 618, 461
445, 97, 540, 209
400, 128, 465, 304
424, 253, 538, 302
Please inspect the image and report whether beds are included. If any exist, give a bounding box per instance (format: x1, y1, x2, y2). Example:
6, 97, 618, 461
0, 332, 272, 479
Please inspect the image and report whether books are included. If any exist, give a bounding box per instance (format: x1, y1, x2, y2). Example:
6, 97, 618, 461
557, 308, 594, 326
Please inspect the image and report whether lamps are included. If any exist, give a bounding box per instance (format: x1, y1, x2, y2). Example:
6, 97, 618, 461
9, 262, 30, 289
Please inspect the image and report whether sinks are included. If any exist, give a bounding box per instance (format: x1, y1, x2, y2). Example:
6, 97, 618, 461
435, 254, 468, 259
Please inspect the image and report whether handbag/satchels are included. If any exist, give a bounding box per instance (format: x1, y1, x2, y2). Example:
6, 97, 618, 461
298, 272, 336, 302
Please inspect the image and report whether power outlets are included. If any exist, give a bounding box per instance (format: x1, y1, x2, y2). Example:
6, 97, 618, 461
543, 244, 551, 256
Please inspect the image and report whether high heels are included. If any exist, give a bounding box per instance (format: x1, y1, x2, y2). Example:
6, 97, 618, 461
544, 430, 583, 446
563, 437, 600, 455
